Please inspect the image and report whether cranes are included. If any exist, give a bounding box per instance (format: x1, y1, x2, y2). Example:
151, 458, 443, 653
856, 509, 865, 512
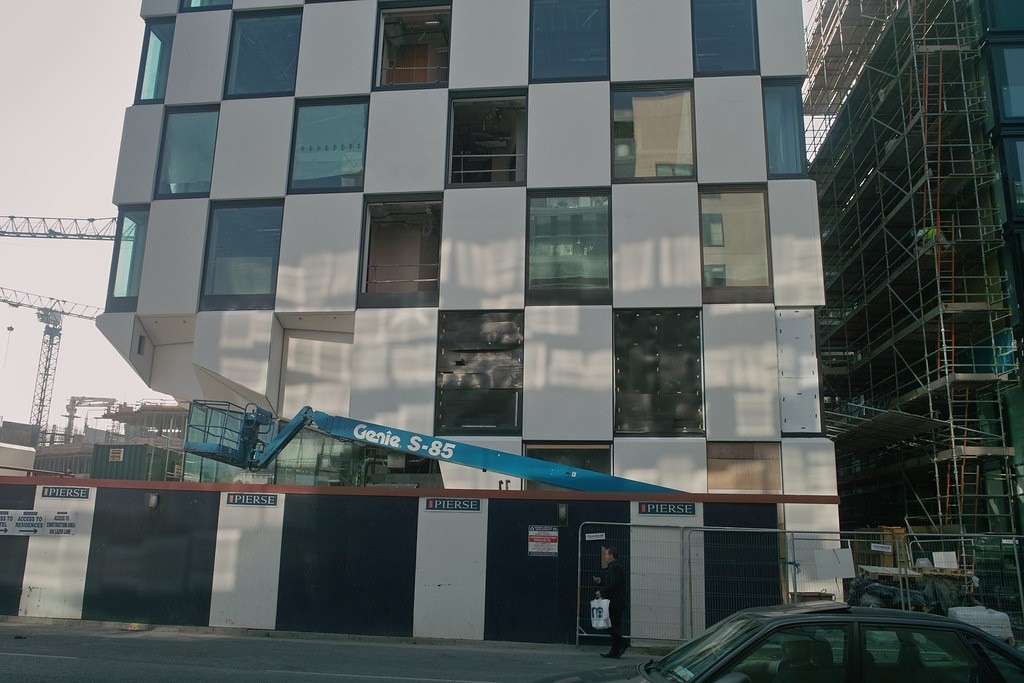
0, 288, 105, 447
0, 214, 136, 243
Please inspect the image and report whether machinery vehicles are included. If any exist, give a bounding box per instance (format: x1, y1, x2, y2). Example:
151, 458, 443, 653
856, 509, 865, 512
181, 399, 687, 493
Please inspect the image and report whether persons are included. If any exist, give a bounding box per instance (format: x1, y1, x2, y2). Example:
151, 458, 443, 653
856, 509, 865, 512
911, 227, 941, 248
594, 547, 627, 658
733, 629, 816, 683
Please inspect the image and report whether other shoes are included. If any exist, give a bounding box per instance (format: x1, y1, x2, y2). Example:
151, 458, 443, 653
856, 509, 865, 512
620, 642, 630, 655
600, 654, 620, 659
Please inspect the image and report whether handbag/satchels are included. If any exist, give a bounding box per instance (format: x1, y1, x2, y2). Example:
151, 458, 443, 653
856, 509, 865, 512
590, 595, 612, 630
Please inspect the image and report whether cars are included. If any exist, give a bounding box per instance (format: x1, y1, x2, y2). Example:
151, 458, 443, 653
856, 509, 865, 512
624, 600, 1024, 683
